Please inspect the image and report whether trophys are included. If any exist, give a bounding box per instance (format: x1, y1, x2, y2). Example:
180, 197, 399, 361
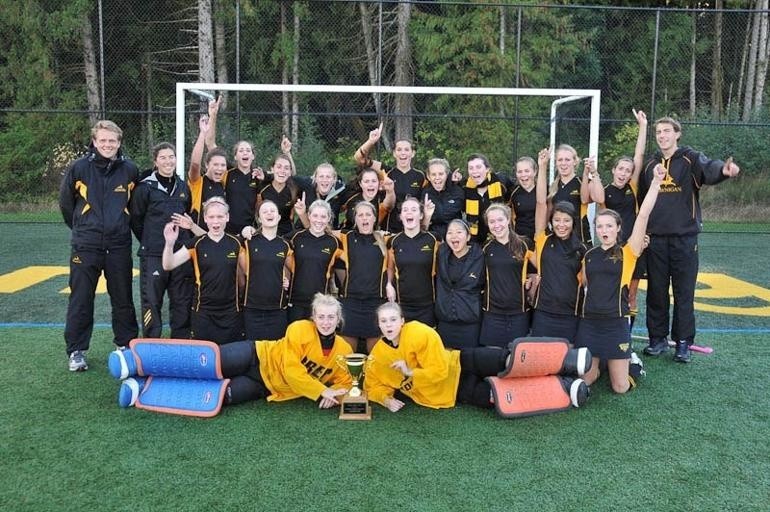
335, 350, 376, 421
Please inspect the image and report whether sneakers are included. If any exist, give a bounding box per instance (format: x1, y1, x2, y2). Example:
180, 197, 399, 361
630, 358, 646, 377
108, 348, 147, 408
644, 339, 668, 356
561, 347, 592, 407
69, 351, 88, 371
673, 340, 691, 362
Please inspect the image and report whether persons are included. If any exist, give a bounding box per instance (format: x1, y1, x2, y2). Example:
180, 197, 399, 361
108, 292, 356, 417
163, 91, 604, 344
583, 108, 649, 338
364, 301, 590, 419
644, 119, 740, 364
129, 142, 196, 338
579, 164, 668, 392
59, 121, 140, 372
